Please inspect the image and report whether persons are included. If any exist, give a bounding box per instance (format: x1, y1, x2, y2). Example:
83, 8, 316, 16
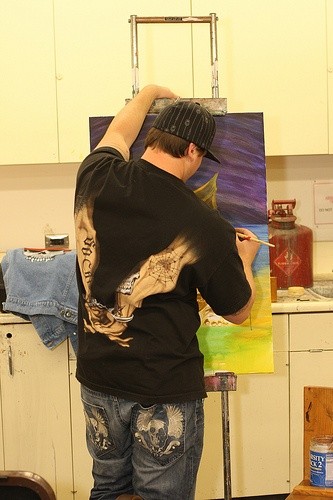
74, 83, 263, 500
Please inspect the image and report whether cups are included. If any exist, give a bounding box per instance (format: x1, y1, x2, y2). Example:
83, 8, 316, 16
309, 435, 333, 488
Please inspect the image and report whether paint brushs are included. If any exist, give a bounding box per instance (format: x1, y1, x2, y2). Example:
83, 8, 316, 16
237, 232, 276, 248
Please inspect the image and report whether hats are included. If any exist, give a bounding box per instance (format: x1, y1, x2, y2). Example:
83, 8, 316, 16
153, 99, 226, 166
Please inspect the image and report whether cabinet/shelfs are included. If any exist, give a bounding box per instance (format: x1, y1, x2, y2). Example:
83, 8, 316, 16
0, 0, 333, 165
0, 311, 333, 500
